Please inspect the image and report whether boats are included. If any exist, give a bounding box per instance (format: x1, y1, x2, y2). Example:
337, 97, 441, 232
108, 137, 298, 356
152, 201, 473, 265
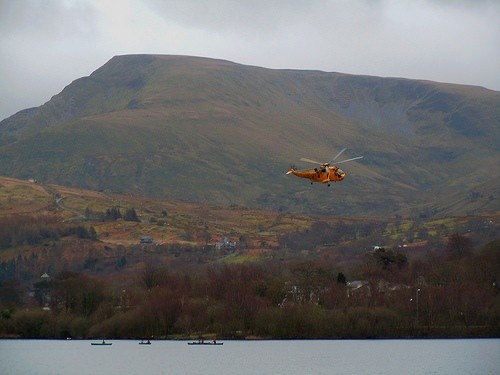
91, 340, 113, 345
188, 339, 223, 345
139, 339, 151, 345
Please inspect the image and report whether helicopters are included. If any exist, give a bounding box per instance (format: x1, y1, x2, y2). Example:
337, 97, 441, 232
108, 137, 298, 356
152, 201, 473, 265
285, 148, 364, 187
